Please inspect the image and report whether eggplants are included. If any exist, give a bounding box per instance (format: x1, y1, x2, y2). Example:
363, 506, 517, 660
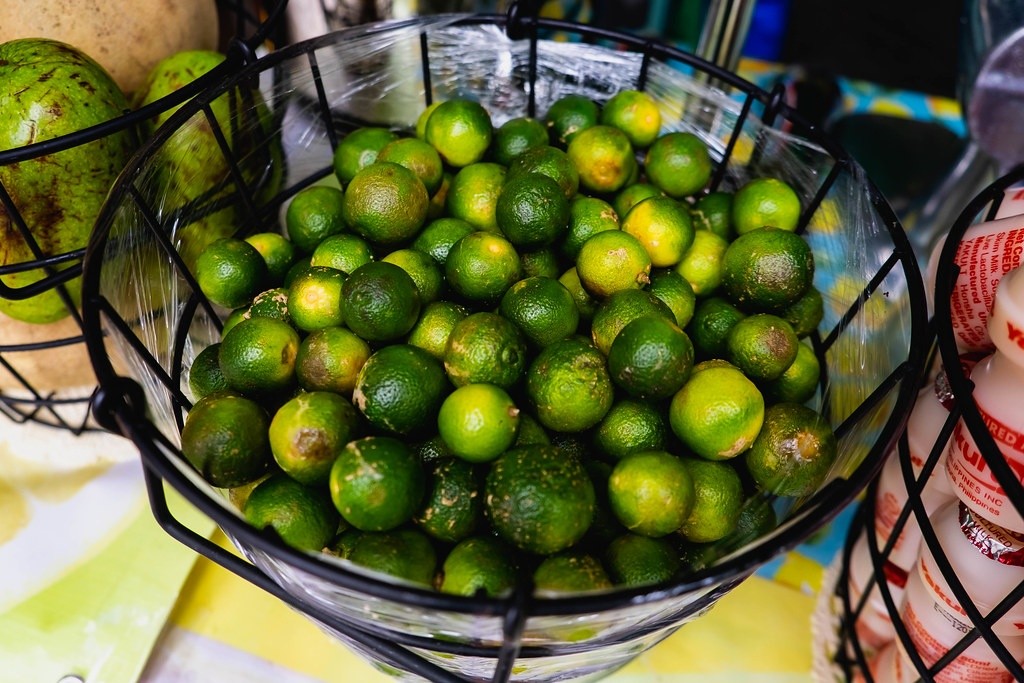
133, 53, 281, 259
0, 38, 142, 323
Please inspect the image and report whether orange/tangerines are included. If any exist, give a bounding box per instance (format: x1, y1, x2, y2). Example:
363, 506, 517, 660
183, 87, 839, 678
